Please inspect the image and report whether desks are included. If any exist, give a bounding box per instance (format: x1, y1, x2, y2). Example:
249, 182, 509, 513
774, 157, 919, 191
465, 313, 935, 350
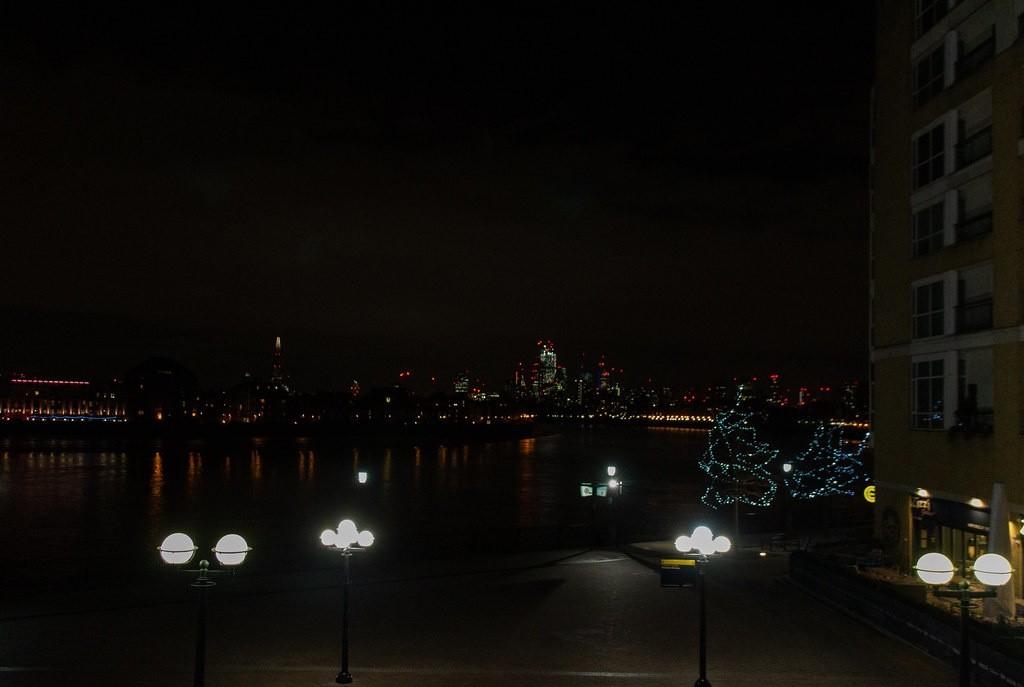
771, 532, 801, 551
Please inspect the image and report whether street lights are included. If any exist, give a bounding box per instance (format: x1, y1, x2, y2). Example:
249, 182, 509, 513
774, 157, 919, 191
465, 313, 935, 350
319, 518, 375, 684
912, 551, 1017, 687
674, 525, 731, 687
156, 531, 252, 687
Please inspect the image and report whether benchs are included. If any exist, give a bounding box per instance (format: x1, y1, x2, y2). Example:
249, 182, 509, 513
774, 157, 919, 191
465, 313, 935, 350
760, 536, 816, 552
